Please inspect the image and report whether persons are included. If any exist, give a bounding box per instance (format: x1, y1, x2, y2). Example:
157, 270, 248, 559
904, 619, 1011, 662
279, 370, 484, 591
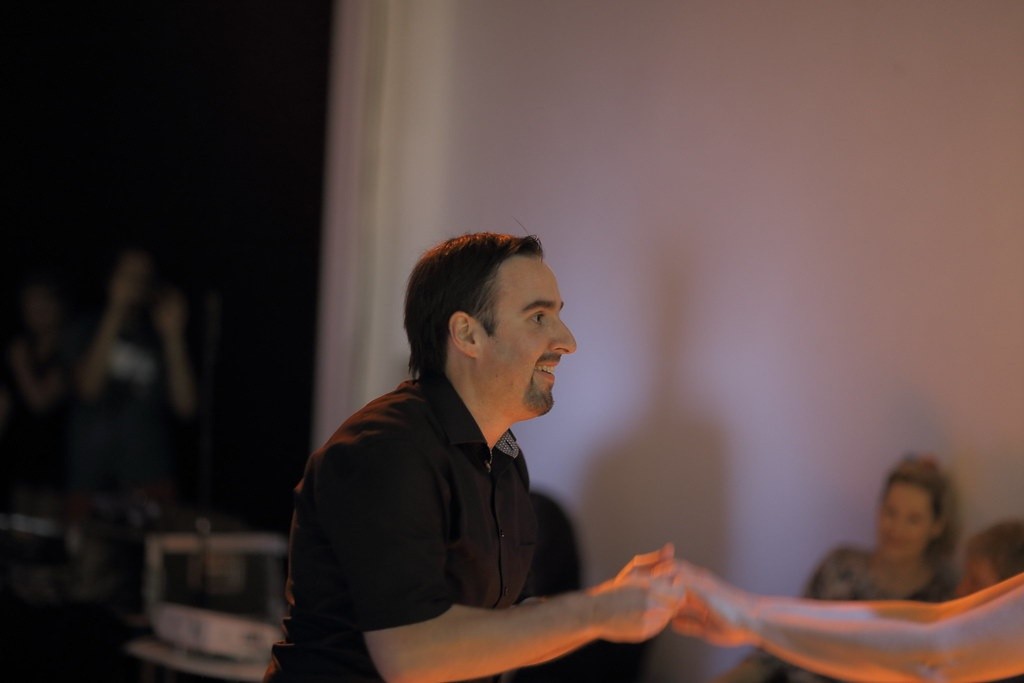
0, 245, 195, 600
711, 453, 1024, 683
650, 560, 1023, 683
260, 231, 686, 683
